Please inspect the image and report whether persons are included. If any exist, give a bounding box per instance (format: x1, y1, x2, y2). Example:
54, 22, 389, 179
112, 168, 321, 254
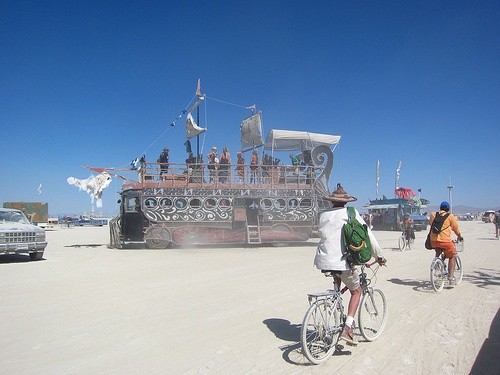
360, 210, 398, 231
427, 201, 463, 281
157, 148, 169, 181
235, 152, 247, 182
185, 154, 205, 182
313, 186, 388, 344
288, 152, 303, 173
400, 213, 415, 245
249, 150, 260, 182
208, 147, 232, 183
493, 209, 500, 237
139, 159, 146, 182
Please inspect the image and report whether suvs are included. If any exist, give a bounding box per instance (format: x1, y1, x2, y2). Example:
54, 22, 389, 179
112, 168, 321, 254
1, 207, 47, 262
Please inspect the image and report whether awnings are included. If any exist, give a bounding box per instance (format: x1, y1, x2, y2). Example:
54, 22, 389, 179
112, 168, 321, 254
263, 130, 342, 165
262, 154, 280, 179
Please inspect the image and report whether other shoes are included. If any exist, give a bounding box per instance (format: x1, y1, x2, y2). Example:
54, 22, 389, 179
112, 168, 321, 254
448, 275, 455, 281
332, 295, 344, 308
340, 325, 358, 345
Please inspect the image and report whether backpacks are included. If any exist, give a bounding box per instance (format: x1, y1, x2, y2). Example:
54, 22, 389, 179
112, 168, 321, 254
341, 206, 374, 264
432, 212, 450, 233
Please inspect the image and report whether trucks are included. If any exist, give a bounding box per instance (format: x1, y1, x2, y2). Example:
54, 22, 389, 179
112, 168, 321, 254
116, 162, 318, 246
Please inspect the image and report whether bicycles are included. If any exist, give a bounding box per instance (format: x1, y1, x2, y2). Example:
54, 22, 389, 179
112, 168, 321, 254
398, 225, 415, 252
298, 260, 389, 364
431, 240, 464, 293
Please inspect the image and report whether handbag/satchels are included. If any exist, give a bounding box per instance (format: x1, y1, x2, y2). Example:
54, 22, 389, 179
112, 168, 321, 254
425, 233, 436, 249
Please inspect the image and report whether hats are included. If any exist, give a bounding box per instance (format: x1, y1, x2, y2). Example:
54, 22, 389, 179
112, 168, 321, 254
441, 202, 450, 209
403, 212, 411, 216
322, 183, 357, 202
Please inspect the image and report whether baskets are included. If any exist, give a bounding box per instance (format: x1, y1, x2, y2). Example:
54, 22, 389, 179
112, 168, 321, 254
453, 239, 464, 252
